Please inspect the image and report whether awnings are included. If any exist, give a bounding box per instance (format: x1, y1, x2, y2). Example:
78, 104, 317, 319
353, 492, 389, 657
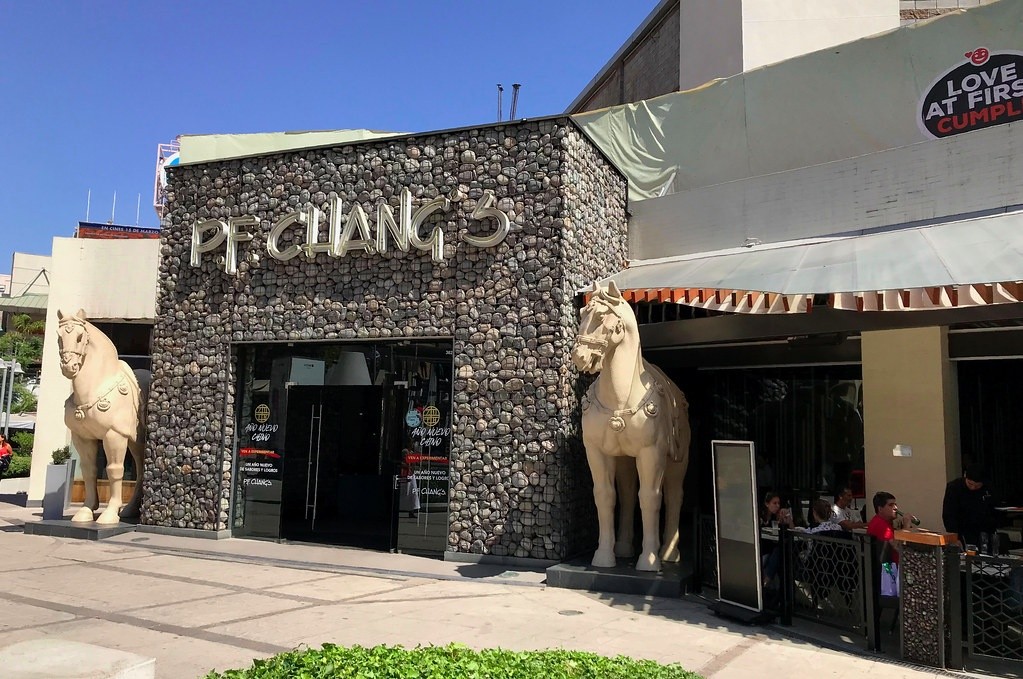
574, 209, 1023, 317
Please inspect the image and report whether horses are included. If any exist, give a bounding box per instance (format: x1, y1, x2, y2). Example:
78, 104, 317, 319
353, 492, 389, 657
58, 308, 152, 525
570, 278, 691, 572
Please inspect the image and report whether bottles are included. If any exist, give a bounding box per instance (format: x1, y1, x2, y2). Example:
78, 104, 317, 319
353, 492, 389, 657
896, 510, 920, 526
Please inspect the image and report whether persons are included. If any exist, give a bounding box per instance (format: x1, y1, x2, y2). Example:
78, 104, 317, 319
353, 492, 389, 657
399, 437, 421, 517
793, 501, 844, 570
759, 493, 794, 579
0, 433, 12, 481
867, 492, 916, 568
942, 459, 998, 552
830, 486, 870, 545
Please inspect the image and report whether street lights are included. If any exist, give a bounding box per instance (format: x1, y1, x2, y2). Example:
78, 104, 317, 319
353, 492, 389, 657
0, 358, 25, 440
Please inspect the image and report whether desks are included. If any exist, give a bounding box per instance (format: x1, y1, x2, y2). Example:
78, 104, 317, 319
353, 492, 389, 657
960, 552, 1021, 614
761, 526, 813, 608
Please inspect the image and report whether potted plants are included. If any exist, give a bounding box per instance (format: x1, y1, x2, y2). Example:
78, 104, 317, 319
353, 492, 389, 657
43, 449, 68, 520
63, 446, 77, 510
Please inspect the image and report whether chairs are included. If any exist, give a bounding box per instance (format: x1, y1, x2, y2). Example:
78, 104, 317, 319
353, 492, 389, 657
850, 532, 901, 637
799, 530, 855, 615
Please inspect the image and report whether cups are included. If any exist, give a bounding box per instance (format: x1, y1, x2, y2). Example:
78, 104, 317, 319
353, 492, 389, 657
772, 521, 779, 535
967, 545, 975, 555
